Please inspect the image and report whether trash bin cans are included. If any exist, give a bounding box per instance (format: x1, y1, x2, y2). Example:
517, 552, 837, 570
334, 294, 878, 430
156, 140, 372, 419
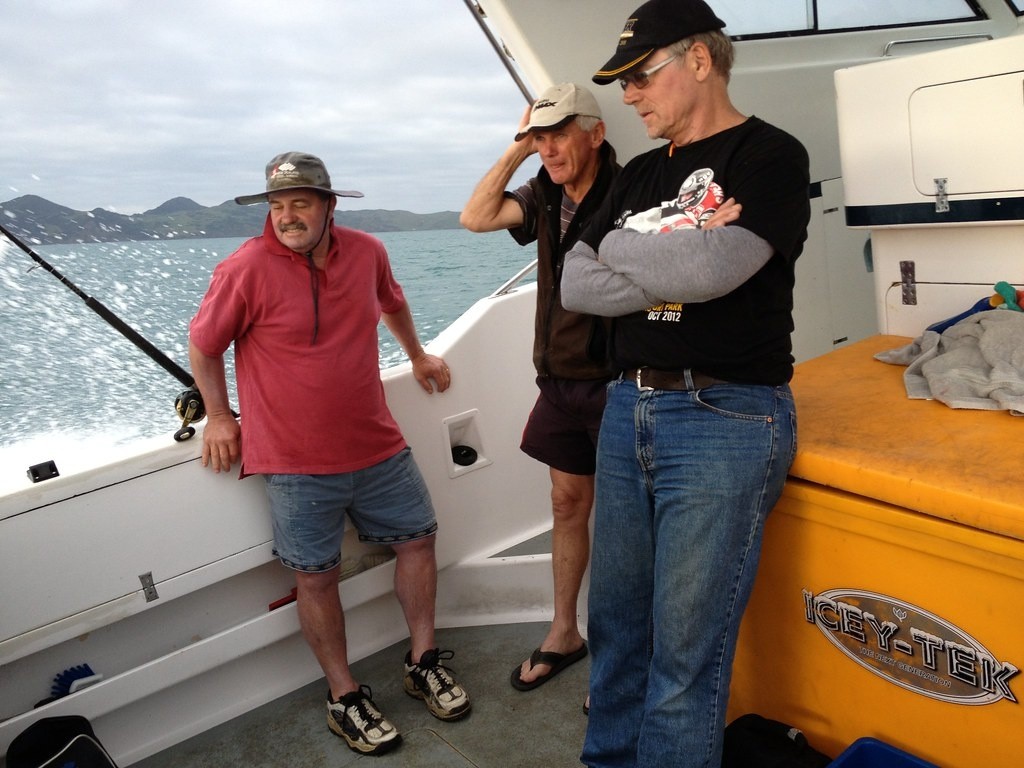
717, 333, 1024, 765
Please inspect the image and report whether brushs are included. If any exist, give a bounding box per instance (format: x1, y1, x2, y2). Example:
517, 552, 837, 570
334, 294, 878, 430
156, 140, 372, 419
48, 662, 104, 698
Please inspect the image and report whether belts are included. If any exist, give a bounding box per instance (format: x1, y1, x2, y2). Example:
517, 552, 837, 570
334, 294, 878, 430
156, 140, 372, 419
621, 364, 730, 392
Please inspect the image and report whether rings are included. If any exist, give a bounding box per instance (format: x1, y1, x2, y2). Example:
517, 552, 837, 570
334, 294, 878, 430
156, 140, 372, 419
446, 369, 448, 371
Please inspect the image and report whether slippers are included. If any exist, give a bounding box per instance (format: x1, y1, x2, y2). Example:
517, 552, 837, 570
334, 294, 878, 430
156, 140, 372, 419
510, 641, 589, 693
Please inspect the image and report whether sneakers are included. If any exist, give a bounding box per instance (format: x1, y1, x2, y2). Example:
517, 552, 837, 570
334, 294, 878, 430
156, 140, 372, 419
403, 647, 473, 724
326, 683, 403, 756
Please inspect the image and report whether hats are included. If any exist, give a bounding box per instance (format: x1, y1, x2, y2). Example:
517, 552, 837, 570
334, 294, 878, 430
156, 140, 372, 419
235, 150, 366, 207
592, 1, 726, 85
514, 81, 602, 143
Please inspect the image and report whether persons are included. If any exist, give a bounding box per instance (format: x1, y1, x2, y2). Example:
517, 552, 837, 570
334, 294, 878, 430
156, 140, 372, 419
559, 0, 811, 768
188, 151, 471, 757
459, 81, 623, 716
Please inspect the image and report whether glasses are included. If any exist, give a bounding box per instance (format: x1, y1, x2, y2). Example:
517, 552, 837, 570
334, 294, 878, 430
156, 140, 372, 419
618, 55, 683, 89
